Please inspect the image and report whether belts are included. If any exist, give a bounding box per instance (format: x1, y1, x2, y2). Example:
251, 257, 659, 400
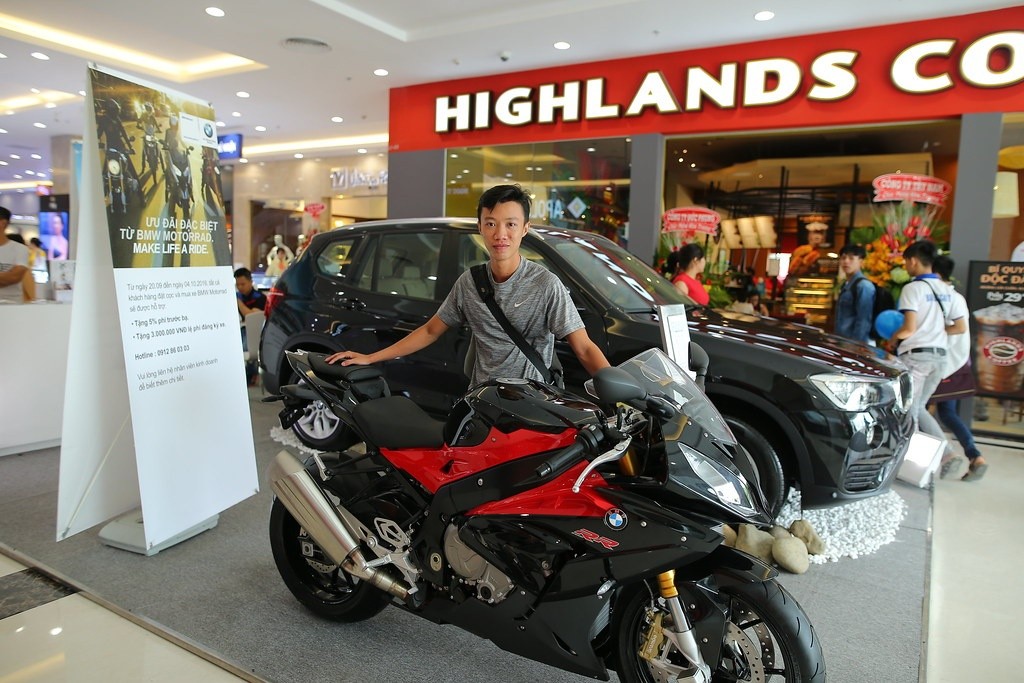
900, 347, 946, 357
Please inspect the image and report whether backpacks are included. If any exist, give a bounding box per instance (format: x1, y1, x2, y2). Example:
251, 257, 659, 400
851, 277, 895, 340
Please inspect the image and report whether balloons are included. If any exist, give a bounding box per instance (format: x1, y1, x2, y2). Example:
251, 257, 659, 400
877, 310, 906, 342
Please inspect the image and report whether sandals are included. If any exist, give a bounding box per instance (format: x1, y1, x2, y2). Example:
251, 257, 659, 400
962, 456, 988, 481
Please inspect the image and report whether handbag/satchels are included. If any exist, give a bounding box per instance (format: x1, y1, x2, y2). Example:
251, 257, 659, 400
928, 363, 976, 402
464, 332, 565, 390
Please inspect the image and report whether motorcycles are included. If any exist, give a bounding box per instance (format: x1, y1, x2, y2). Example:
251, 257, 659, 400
267, 346, 827, 683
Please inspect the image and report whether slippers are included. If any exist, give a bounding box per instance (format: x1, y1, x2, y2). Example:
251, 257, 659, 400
940, 455, 962, 480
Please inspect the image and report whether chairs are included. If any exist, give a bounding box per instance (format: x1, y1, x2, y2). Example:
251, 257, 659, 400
361, 259, 437, 299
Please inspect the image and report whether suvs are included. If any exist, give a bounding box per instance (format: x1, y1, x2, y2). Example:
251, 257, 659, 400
256, 215, 917, 514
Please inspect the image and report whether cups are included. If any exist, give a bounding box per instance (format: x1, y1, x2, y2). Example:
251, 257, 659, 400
973, 308, 1024, 396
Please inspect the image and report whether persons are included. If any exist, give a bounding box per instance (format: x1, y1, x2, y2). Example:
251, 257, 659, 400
163, 116, 195, 203
888, 240, 967, 480
136, 102, 165, 175
265, 248, 291, 276
296, 234, 306, 257
267, 234, 294, 268
233, 268, 267, 352
28, 237, 46, 260
48, 216, 68, 261
201, 145, 223, 202
324, 185, 612, 391
0, 205, 30, 305
834, 245, 875, 344
747, 290, 769, 317
666, 243, 710, 306
96, 99, 147, 209
925, 255, 988, 482
5, 234, 37, 303
